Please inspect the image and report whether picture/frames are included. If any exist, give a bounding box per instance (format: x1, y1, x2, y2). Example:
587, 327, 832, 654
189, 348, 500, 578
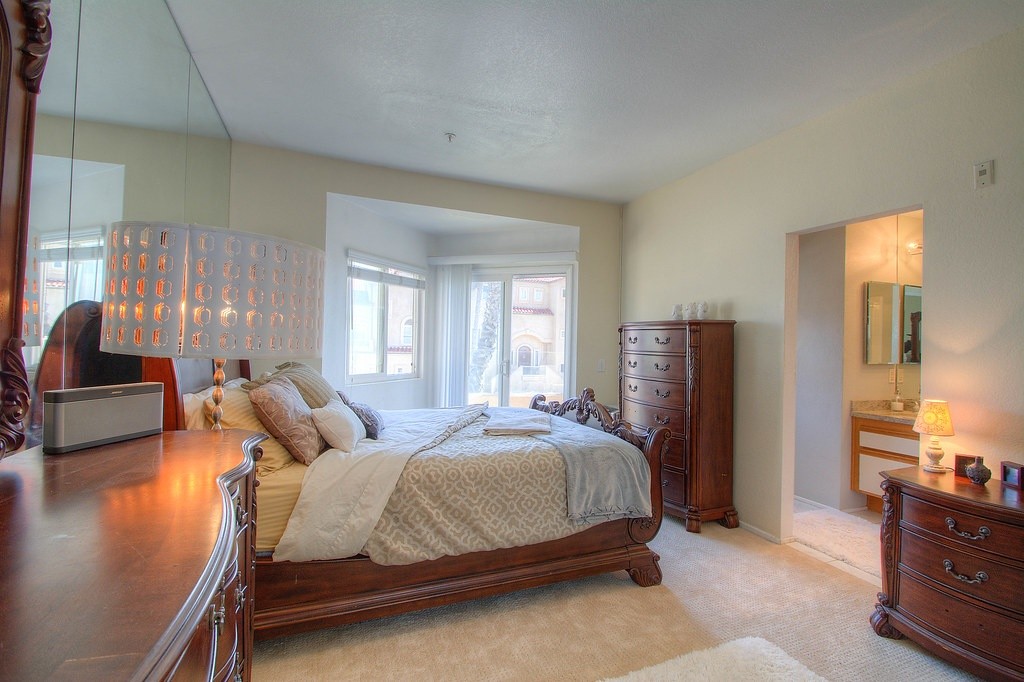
952, 454, 983, 477
1000, 461, 1024, 490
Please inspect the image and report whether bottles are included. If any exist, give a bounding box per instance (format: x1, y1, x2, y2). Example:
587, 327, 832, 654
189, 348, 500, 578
965, 457, 991, 486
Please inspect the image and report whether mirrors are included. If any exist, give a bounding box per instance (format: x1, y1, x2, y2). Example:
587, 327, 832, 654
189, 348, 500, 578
903, 284, 924, 365
22, 1, 236, 443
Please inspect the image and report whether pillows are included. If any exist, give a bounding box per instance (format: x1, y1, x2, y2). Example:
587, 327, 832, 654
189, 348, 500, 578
181, 358, 383, 478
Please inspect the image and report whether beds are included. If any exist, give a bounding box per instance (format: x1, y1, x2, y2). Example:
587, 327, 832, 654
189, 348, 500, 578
174, 348, 666, 635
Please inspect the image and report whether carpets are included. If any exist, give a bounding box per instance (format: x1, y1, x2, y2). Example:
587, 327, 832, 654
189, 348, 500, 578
599, 636, 825, 682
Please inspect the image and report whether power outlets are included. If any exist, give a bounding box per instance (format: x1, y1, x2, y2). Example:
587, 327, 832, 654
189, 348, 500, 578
889, 368, 896, 382
897, 369, 905, 383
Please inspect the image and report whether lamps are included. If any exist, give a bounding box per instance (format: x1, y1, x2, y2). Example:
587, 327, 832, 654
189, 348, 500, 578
98, 221, 180, 357
181, 220, 325, 427
910, 399, 955, 473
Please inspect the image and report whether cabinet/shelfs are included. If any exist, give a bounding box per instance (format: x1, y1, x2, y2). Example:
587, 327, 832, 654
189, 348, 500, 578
615, 318, 739, 533
871, 464, 1024, 682
851, 419, 916, 513
1, 430, 269, 682
